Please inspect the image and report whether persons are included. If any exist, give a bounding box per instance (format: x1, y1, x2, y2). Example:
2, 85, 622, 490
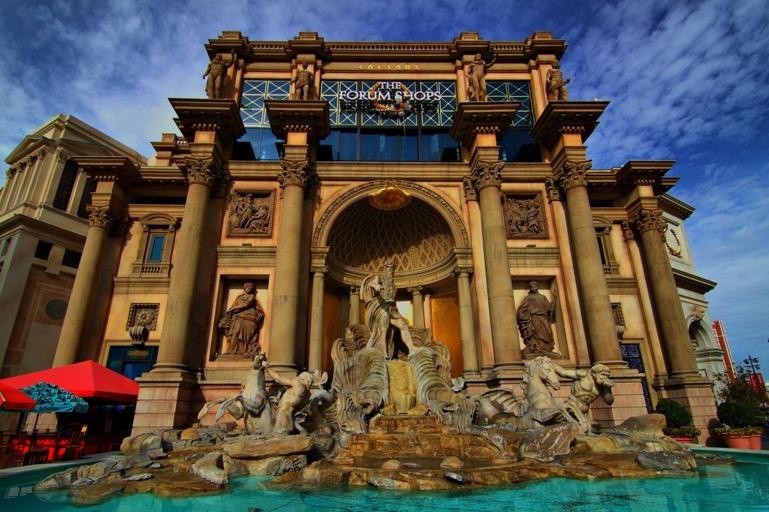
202, 48, 236, 98
504, 196, 544, 236
462, 50, 500, 101
261, 360, 314, 437
218, 281, 265, 356
229, 193, 270, 234
553, 360, 615, 436
359, 274, 421, 361
288, 60, 314, 102
545, 58, 569, 101
515, 280, 559, 355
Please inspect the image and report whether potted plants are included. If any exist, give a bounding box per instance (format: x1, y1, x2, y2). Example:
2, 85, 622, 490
651, 357, 766, 451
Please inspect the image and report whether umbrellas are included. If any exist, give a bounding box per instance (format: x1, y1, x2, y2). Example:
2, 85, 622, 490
16, 380, 90, 441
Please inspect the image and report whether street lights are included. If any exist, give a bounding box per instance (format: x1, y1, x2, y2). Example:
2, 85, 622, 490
741, 352, 766, 408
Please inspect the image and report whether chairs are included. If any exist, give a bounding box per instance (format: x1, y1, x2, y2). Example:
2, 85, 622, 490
0, 432, 118, 497
